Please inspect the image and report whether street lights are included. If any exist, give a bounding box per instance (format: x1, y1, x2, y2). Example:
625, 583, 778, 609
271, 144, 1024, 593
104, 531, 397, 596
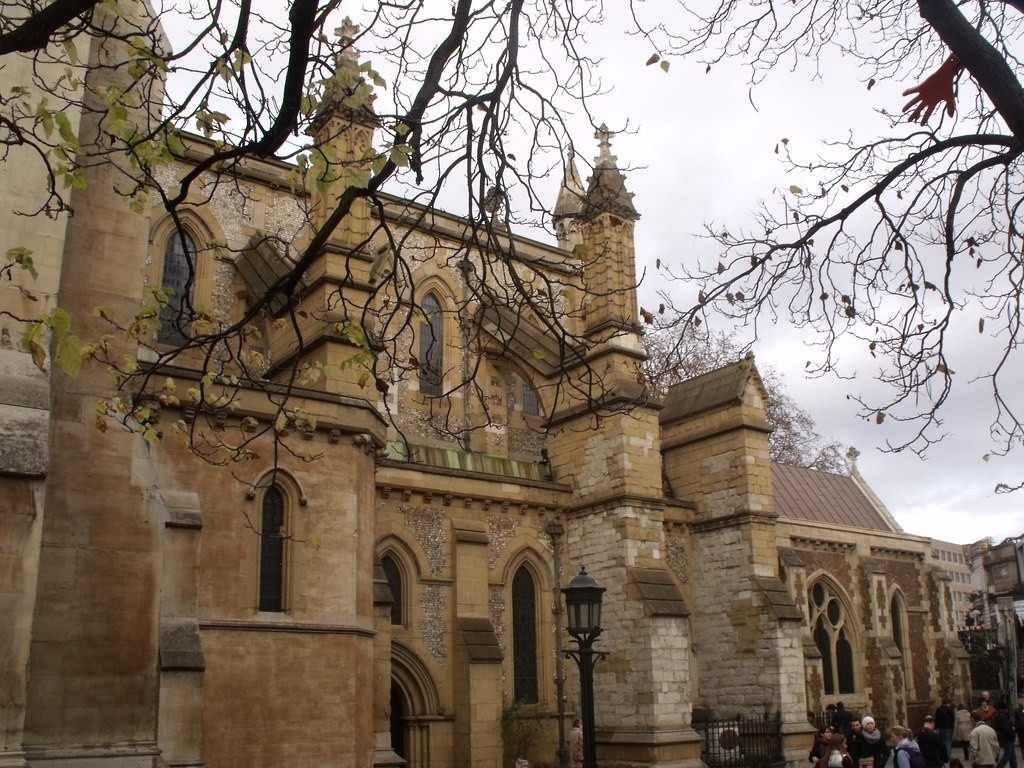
555, 567, 609, 767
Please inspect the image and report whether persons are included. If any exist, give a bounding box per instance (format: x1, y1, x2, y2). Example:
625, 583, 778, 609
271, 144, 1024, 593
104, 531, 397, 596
809, 691, 950, 768
936, 691, 1024, 768
808, 702, 853, 732
568, 718, 583, 768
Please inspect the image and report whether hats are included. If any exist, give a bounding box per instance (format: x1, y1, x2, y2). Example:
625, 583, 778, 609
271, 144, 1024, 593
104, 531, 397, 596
862, 716, 875, 728
